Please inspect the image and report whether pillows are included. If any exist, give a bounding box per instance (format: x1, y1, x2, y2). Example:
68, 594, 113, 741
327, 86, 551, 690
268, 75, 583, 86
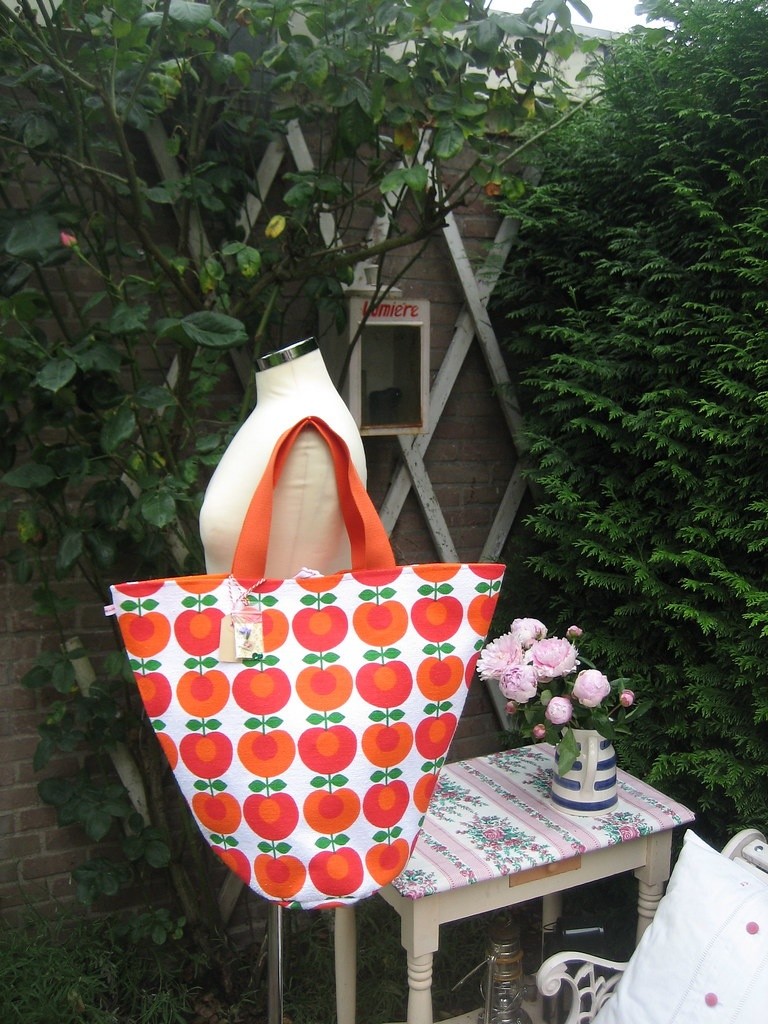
590, 829, 768, 1024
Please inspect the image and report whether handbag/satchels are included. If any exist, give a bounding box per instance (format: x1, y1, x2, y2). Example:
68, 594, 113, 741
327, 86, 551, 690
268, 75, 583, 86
105, 414, 505, 910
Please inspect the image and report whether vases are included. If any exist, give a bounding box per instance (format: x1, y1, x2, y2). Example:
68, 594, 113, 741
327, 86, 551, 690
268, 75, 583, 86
549, 717, 618, 817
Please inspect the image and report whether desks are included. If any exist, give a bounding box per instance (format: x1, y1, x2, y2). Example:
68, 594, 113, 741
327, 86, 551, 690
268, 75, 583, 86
334, 740, 695, 1024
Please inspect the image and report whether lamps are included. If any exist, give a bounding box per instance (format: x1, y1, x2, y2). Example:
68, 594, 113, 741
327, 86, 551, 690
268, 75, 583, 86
450, 909, 533, 1024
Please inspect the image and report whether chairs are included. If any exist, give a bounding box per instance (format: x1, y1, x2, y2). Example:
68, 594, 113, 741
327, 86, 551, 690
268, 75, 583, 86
536, 829, 768, 1024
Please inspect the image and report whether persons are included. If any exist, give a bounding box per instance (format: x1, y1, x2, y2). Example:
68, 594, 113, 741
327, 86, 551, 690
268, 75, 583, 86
198, 336, 370, 585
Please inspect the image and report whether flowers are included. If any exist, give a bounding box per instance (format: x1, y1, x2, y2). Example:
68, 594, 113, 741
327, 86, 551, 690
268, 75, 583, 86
476, 618, 655, 776
